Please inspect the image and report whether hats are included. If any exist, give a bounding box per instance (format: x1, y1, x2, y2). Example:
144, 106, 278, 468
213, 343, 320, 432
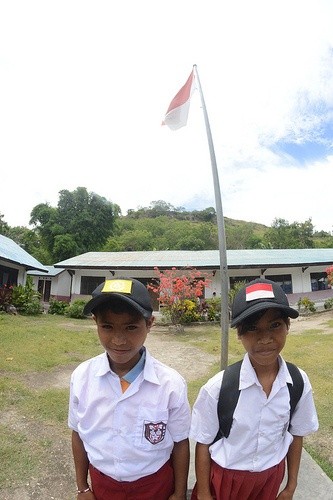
82, 276, 153, 321
230, 278, 299, 328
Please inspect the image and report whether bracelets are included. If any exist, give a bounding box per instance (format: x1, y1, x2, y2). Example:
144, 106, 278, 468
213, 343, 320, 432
76, 488, 90, 493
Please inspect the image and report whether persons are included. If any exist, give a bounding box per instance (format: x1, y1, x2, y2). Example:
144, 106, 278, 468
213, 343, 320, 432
189, 278, 319, 500
68, 277, 191, 500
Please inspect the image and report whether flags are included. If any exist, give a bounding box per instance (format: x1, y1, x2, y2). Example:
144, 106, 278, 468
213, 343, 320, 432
161, 67, 202, 139
